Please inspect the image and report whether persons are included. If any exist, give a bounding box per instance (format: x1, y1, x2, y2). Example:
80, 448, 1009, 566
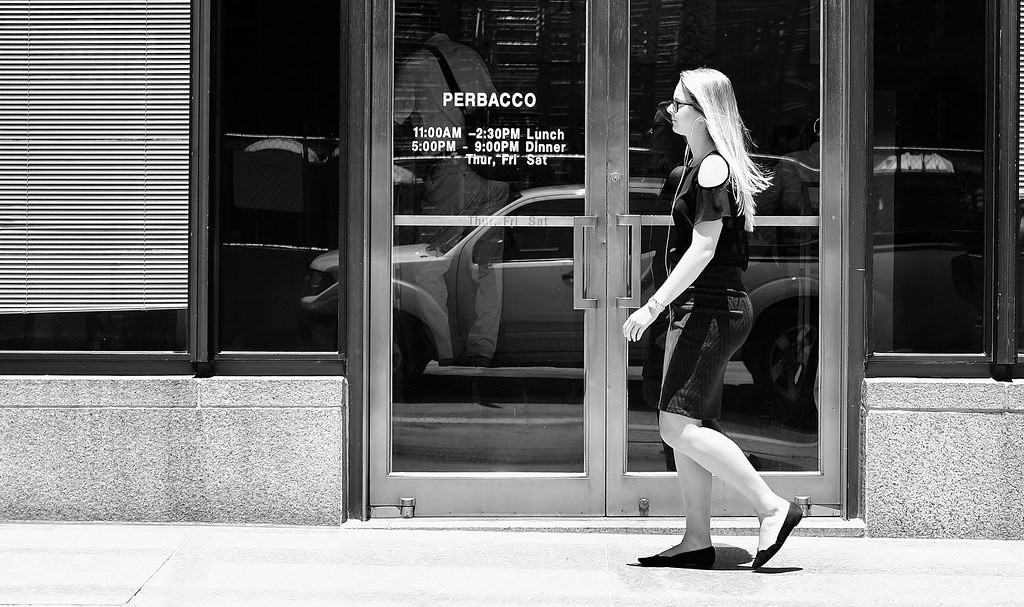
620, 67, 804, 571
393, 0, 510, 367
646, 100, 686, 289
754, 120, 819, 259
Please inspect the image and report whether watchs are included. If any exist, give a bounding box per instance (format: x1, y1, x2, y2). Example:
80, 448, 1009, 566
648, 296, 665, 312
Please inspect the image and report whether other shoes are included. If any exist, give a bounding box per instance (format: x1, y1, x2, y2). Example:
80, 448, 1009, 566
752, 501, 803, 569
638, 545, 715, 570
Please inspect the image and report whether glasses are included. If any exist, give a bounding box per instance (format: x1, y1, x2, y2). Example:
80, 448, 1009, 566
673, 101, 694, 111
653, 123, 668, 130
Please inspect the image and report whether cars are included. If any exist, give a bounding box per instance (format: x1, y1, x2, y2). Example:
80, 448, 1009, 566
293, 173, 818, 435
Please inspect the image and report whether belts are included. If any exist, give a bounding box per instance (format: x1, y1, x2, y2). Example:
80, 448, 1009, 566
429, 149, 464, 165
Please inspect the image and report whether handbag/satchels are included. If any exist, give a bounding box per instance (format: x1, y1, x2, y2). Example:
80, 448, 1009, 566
463, 106, 542, 181
